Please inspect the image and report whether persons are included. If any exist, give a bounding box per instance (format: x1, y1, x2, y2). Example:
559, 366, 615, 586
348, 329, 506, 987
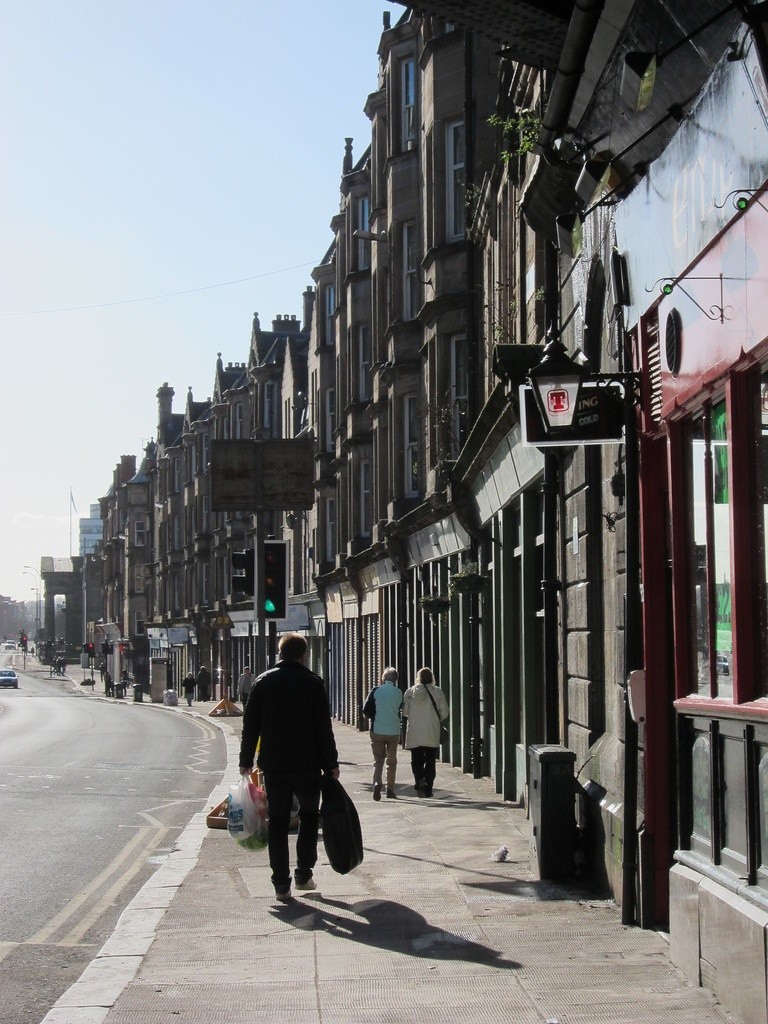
24, 646, 28, 656
238, 666, 254, 707
196, 666, 211, 702
31, 648, 34, 657
402, 668, 450, 796
182, 672, 196, 706
99, 663, 106, 682
241, 633, 340, 900
52, 655, 67, 676
363, 667, 404, 802
106, 672, 111, 690
121, 670, 128, 697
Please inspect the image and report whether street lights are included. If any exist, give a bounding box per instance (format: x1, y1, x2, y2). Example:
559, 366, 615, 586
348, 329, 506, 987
22, 571, 38, 656
24, 565, 42, 630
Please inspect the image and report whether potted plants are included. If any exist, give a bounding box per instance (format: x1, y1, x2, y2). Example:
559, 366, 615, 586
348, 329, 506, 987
449, 561, 489, 594
420, 594, 450, 612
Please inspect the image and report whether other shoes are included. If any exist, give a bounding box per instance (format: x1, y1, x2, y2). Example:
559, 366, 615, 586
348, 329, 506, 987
425, 788, 433, 798
387, 789, 396, 798
372, 781, 381, 801
418, 779, 425, 798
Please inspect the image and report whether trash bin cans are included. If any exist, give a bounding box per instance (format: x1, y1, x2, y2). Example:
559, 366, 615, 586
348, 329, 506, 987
116, 683, 123, 699
132, 684, 143, 702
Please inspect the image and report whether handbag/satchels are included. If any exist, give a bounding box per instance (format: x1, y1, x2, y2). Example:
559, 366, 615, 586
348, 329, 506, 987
245, 773, 266, 819
227, 772, 270, 851
319, 772, 363, 875
440, 725, 448, 745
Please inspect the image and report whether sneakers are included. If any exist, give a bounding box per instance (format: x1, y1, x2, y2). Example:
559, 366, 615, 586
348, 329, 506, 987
276, 888, 291, 900
295, 878, 317, 890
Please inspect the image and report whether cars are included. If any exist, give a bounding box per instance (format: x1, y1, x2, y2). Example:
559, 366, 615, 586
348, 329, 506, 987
0, 669, 19, 689
4, 639, 16, 650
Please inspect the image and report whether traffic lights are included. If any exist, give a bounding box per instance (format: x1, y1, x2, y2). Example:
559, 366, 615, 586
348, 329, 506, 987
84, 642, 94, 657
264, 539, 292, 622
231, 546, 254, 597
22, 636, 27, 647
